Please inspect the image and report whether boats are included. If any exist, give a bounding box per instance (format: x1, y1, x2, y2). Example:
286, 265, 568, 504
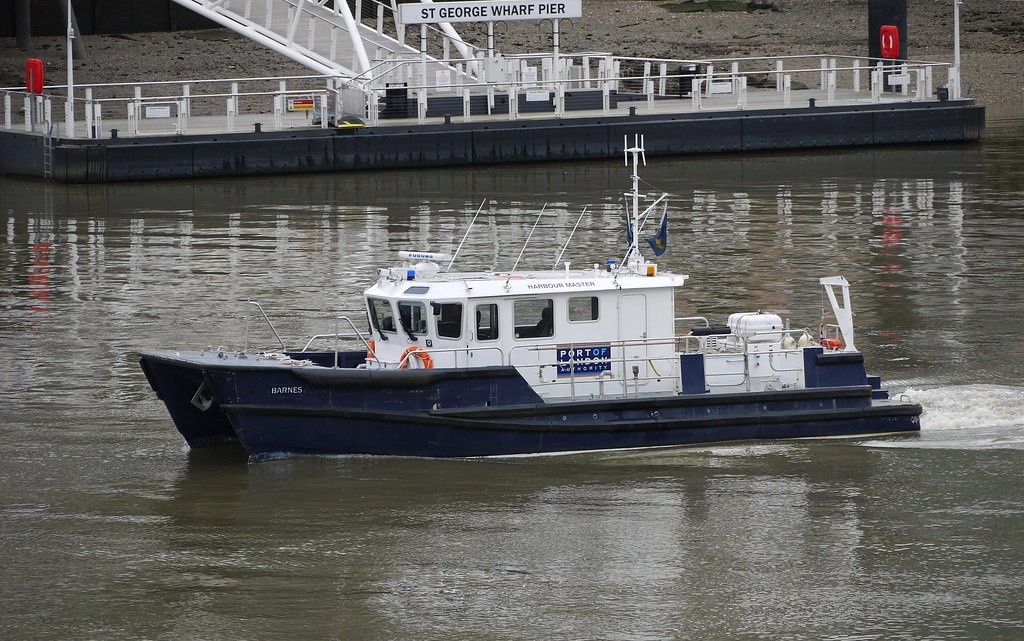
136, 134, 923, 462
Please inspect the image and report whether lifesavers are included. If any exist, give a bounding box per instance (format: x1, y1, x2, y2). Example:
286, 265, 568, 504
399, 346, 433, 369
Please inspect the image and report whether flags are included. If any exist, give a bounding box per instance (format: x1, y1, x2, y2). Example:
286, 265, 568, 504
645, 198, 667, 257
624, 196, 633, 247
